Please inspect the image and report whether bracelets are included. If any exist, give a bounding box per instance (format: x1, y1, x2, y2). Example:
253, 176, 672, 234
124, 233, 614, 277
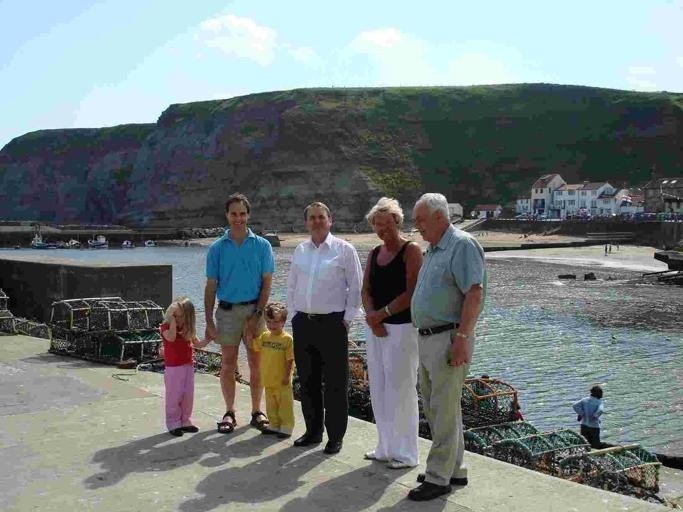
384, 305, 393, 318
455, 331, 468, 339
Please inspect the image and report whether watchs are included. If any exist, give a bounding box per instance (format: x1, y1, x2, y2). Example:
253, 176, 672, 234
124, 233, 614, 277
254, 308, 263, 316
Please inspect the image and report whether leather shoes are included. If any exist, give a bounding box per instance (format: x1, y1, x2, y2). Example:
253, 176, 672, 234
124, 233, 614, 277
170, 411, 467, 501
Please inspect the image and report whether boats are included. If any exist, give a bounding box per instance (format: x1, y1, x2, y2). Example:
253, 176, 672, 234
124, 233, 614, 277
29, 233, 48, 249
143, 239, 157, 248
55, 238, 79, 248
12, 244, 21, 248
121, 239, 135, 248
87, 234, 108, 249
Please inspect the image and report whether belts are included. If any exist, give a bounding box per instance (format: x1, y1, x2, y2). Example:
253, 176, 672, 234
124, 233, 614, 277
240, 300, 260, 305
418, 322, 459, 336
300, 312, 338, 320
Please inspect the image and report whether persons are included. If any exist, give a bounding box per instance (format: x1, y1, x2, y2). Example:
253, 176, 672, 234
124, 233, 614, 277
473, 374, 496, 394
245, 303, 294, 439
158, 297, 209, 436
573, 387, 604, 449
508, 401, 524, 421
287, 201, 363, 454
204, 194, 276, 433
362, 197, 424, 470
408, 190, 486, 502
